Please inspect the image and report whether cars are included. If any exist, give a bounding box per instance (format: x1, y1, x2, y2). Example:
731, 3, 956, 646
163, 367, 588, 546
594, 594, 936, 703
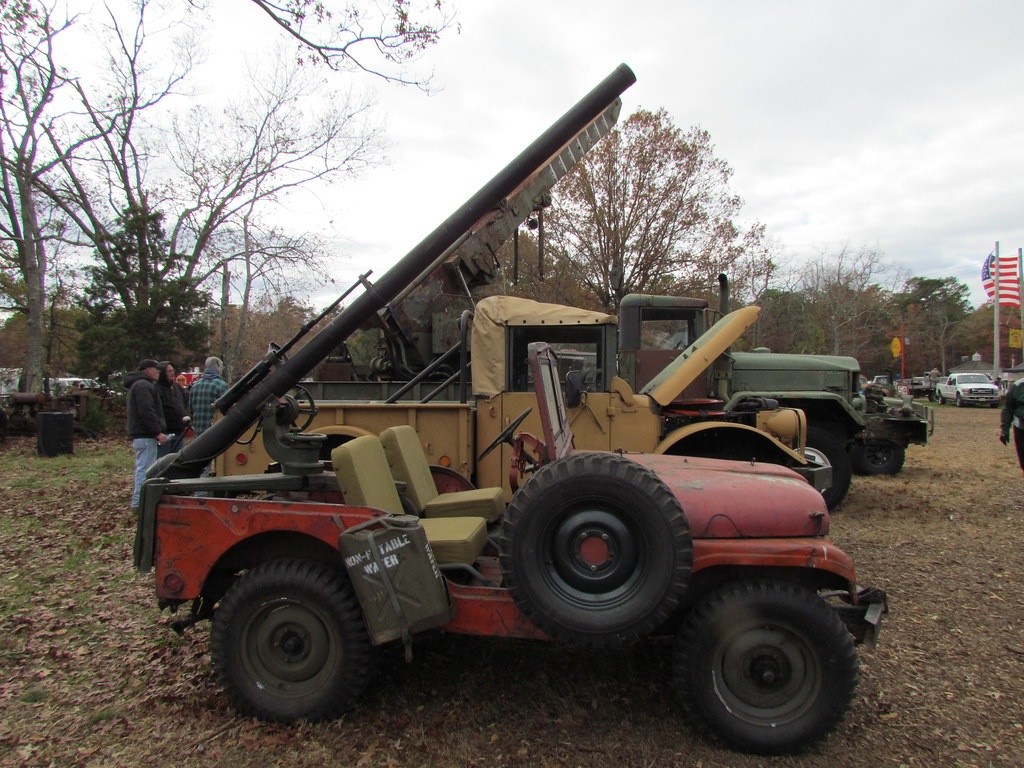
9, 379, 120, 415
860, 385, 934, 475
869, 375, 888, 388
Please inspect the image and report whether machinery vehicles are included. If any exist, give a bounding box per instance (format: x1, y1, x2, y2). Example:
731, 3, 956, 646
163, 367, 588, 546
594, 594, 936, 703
288, 95, 865, 513
130, 53, 889, 755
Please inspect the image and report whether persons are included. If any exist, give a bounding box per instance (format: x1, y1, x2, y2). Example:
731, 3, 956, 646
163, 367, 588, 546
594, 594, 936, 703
929, 368, 940, 386
999, 378, 1024, 473
122, 357, 230, 508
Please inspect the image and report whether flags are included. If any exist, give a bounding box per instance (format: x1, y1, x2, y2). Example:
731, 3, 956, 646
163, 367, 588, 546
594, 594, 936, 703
982, 253, 1020, 306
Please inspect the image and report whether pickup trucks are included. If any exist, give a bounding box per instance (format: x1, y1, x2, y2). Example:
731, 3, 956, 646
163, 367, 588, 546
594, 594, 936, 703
935, 373, 1000, 408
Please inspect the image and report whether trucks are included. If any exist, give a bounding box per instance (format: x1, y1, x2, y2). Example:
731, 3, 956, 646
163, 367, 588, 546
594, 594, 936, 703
211, 294, 833, 506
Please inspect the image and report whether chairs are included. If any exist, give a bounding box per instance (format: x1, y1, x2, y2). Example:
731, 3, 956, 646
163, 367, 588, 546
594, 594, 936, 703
379, 424, 506, 521
331, 435, 489, 567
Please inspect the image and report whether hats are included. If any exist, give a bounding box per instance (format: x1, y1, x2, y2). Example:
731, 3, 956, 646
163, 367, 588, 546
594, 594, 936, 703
140, 359, 164, 370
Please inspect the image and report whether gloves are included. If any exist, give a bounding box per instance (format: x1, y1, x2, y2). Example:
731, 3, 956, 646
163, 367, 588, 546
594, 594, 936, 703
1000, 433, 1009, 445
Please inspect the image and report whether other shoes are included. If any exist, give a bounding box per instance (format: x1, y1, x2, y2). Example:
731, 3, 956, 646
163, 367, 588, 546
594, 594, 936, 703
128, 510, 138, 521
